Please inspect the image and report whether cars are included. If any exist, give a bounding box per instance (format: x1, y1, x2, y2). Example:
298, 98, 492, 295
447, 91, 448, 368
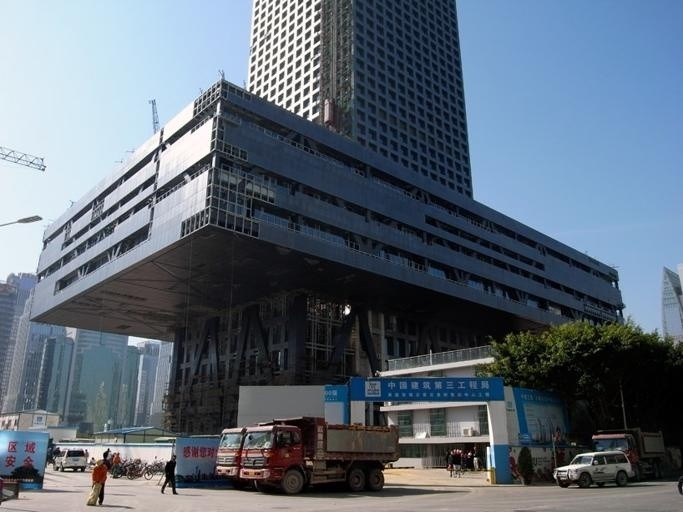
52, 448, 86, 472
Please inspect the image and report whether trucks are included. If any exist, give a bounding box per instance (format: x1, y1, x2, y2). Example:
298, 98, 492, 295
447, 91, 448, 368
590, 428, 665, 481
240, 416, 401, 495
214, 427, 249, 489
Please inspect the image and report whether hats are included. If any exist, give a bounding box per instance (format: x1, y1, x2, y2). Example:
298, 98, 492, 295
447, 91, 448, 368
96, 460, 104, 467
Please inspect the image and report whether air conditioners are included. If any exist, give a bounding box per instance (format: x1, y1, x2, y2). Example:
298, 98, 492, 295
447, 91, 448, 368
461, 427, 473, 437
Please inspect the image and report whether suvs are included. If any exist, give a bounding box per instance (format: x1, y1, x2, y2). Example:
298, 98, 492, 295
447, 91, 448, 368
552, 450, 635, 488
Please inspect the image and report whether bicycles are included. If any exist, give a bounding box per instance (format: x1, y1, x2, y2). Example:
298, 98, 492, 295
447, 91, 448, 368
113, 457, 153, 480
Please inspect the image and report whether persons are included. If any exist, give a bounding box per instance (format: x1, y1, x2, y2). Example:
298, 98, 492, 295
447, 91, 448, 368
86, 459, 108, 505
88, 457, 96, 465
83, 448, 88, 457
98, 451, 110, 505
111, 452, 121, 478
53, 446, 60, 455
101, 447, 117, 471
441, 448, 480, 480
160, 454, 178, 495
